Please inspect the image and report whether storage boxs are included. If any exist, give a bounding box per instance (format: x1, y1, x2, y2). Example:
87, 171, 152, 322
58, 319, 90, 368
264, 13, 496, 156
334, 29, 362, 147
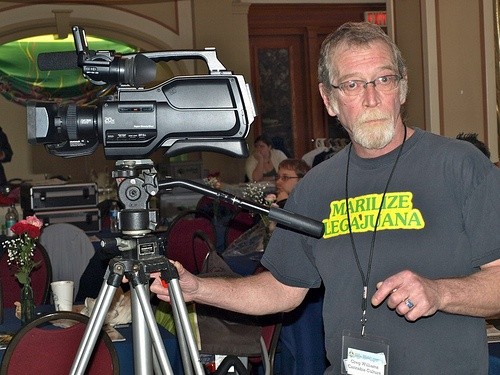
20, 183, 103, 234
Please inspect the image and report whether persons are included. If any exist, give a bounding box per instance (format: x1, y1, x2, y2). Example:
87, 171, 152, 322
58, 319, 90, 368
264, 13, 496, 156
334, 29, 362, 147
140, 18, 500, 374
0, 125, 15, 194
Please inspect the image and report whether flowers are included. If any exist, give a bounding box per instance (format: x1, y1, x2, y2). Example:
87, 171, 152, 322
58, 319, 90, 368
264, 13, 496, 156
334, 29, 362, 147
2, 216, 44, 283
241, 182, 278, 228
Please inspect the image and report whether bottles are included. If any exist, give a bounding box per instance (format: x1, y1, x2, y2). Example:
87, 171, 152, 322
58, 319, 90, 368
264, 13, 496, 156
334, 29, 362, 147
11, 204, 19, 222
110, 202, 120, 233
6, 208, 17, 241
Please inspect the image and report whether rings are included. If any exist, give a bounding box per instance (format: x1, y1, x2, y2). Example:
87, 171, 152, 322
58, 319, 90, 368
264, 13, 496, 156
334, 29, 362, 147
404, 298, 414, 309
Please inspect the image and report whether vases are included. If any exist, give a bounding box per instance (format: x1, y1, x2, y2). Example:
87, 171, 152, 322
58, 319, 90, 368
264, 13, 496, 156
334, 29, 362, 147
22, 283, 33, 328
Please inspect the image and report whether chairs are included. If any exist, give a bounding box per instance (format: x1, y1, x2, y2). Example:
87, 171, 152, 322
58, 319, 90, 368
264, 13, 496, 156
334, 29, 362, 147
0, 310, 119, 375
164, 194, 282, 375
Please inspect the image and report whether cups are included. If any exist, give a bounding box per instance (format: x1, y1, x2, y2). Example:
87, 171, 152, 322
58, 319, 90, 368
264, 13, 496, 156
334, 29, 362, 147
50, 281, 74, 312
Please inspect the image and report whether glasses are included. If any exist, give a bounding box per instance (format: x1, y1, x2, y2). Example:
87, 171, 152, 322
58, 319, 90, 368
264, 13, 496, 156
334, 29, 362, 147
331, 70, 403, 95
275, 175, 300, 181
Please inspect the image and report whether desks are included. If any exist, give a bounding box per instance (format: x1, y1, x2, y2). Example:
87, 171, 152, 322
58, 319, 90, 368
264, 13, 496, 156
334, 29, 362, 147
0, 306, 184, 375
